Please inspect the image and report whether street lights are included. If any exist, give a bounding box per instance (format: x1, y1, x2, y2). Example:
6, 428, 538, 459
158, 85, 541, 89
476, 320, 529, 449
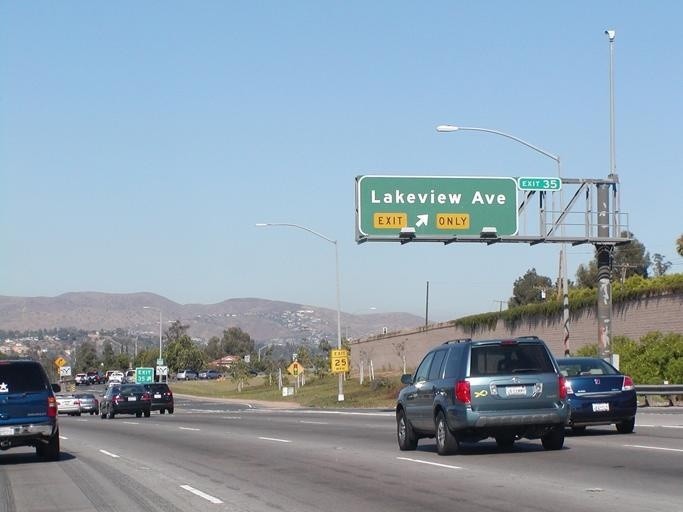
603, 26, 620, 234
140, 305, 163, 382
257, 342, 274, 363
348, 306, 376, 337
254, 221, 346, 405
435, 124, 570, 358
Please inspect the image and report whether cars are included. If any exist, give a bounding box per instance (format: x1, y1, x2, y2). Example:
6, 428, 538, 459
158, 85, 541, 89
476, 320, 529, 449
551, 353, 638, 435
72, 367, 224, 388
53, 392, 81, 416
75, 392, 99, 416
246, 368, 259, 377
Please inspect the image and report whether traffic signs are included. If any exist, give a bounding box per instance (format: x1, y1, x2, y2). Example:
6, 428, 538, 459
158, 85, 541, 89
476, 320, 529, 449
330, 350, 349, 373
516, 177, 563, 193
355, 173, 520, 240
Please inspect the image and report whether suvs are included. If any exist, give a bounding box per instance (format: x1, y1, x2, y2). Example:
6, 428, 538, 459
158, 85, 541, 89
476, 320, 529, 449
139, 381, 174, 415
393, 334, 571, 458
96, 382, 151, 420
0, 357, 65, 463
